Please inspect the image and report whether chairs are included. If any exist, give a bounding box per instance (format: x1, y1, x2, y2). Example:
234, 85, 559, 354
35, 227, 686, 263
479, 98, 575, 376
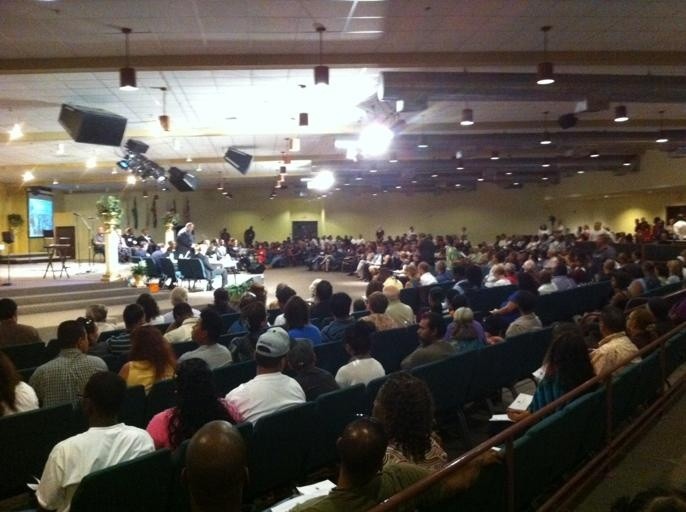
1, 234, 686, 509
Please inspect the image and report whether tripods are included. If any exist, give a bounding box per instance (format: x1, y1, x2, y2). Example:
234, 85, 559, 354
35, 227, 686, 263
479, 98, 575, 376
76, 232, 104, 275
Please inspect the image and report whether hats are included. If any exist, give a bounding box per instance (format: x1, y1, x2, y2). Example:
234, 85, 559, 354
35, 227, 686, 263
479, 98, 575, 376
649, 296, 673, 321
256, 326, 290, 358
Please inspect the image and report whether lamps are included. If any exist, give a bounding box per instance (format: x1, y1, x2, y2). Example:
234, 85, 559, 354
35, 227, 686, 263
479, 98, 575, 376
418, 114, 428, 147
309, 172, 362, 202
119, 29, 138, 91
656, 110, 669, 143
314, 27, 329, 85
614, 98, 628, 122
536, 25, 554, 84
540, 112, 552, 144
159, 89, 170, 131
289, 117, 299, 151
299, 85, 308, 126
460, 68, 474, 126
590, 149, 599, 157
622, 150, 631, 166
369, 149, 552, 195
217, 170, 232, 199
269, 138, 290, 200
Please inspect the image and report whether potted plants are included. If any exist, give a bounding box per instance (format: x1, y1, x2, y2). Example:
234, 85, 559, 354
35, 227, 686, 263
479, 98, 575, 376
93, 192, 120, 234
159, 211, 179, 233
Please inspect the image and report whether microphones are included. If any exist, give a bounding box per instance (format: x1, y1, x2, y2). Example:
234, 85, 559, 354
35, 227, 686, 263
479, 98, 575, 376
88, 217, 94, 219
73, 213, 80, 215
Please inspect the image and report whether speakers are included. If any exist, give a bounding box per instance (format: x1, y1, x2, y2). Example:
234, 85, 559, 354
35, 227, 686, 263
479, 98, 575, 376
248, 263, 266, 273
2, 231, 14, 243
559, 116, 577, 129
